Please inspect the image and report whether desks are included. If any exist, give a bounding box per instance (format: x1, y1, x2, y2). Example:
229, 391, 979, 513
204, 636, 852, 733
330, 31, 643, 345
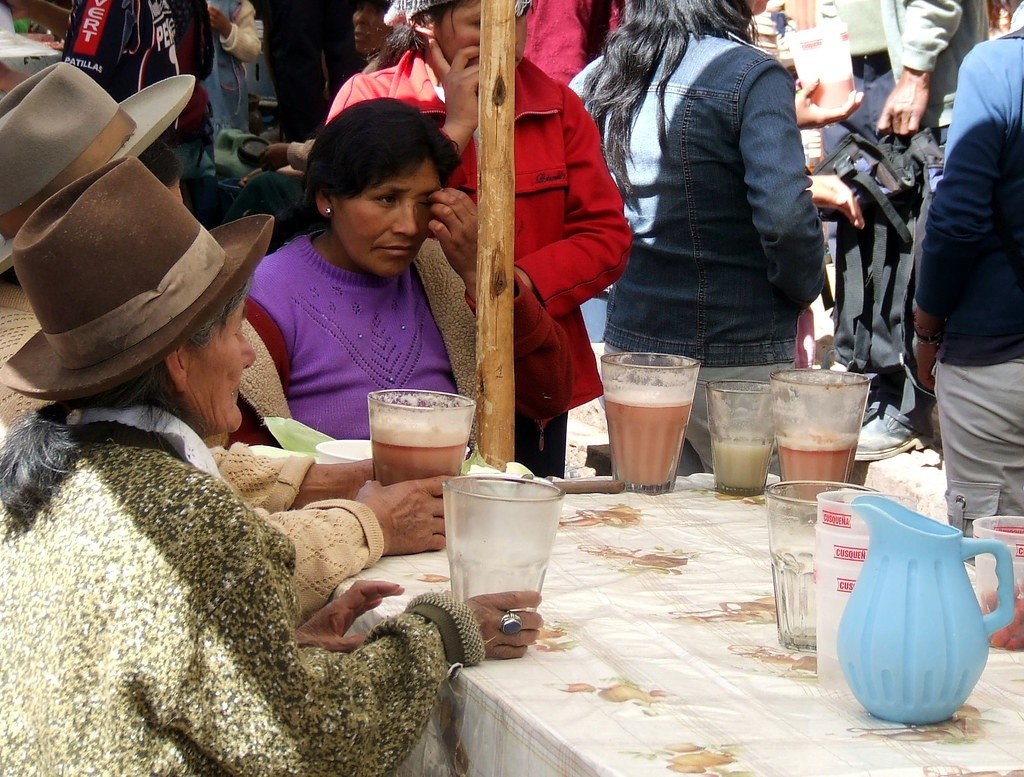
328, 472, 1024, 777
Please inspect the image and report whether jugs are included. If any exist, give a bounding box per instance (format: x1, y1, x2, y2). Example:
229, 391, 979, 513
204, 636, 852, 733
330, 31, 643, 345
836, 493, 1014, 727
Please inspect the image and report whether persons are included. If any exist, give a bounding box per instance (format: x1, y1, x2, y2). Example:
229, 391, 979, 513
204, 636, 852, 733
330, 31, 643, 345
564, 0, 1024, 539
0, 60, 573, 777
63, 0, 635, 480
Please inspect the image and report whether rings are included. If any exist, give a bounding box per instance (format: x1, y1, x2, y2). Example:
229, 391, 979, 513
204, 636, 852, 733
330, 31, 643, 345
499, 611, 523, 635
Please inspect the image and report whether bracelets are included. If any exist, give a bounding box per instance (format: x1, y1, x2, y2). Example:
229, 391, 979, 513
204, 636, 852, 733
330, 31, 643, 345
913, 313, 940, 334
914, 329, 943, 344
916, 339, 938, 345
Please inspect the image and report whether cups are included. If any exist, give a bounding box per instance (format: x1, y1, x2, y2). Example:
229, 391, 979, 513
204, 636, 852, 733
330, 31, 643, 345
815, 488, 919, 692
769, 368, 872, 492
785, 22, 856, 110
705, 378, 776, 497
442, 475, 566, 614
600, 352, 700, 497
972, 516, 1024, 653
764, 480, 880, 654
367, 389, 477, 487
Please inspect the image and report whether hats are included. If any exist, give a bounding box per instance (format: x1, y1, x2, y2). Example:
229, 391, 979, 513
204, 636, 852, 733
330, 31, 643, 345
0, 62, 196, 274
0, 156, 275, 399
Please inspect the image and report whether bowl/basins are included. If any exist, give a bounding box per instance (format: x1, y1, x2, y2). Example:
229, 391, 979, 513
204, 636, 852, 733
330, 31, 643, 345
315, 439, 373, 465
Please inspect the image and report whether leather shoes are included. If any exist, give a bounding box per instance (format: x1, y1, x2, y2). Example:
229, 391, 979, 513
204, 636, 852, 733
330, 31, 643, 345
855, 409, 934, 461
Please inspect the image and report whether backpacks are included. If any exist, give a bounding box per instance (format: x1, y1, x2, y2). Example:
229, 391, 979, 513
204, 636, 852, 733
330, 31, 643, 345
815, 130, 944, 372
